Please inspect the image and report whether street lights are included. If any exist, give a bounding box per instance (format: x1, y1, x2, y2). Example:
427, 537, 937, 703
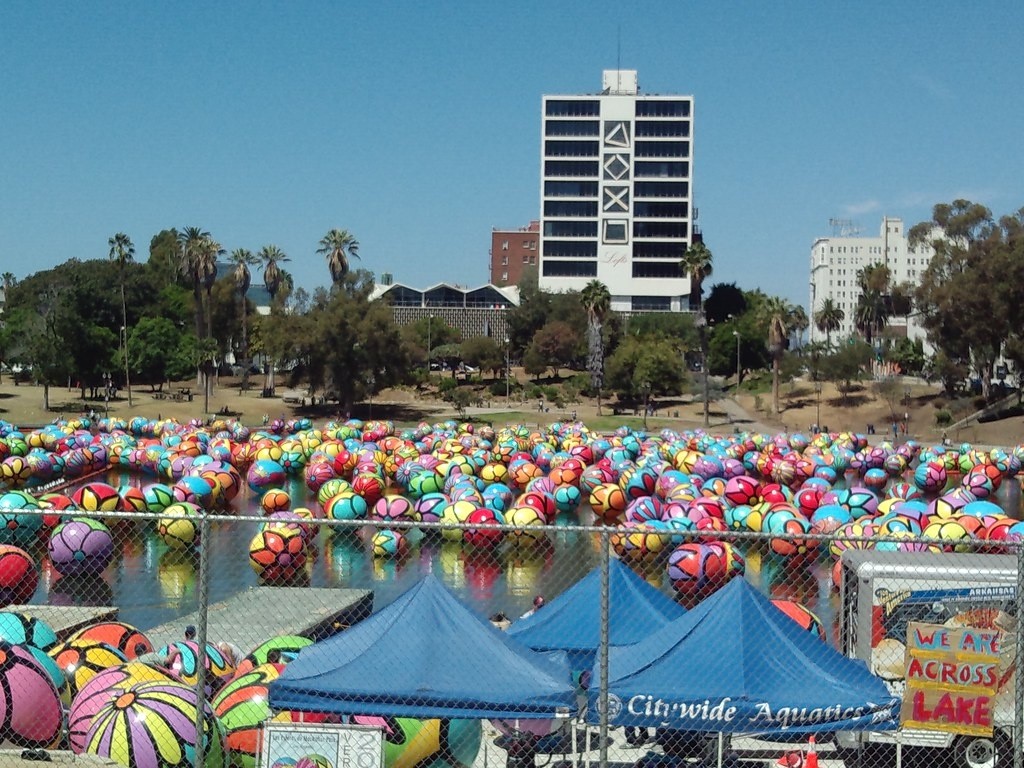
97, 309, 744, 428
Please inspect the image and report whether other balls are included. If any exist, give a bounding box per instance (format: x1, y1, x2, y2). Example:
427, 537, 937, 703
0, 416, 1023, 768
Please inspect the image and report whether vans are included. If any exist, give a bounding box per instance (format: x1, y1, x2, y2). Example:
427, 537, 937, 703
836, 550, 1023, 768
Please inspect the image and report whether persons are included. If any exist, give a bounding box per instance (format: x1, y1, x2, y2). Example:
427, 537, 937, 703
73, 378, 949, 447
217, 642, 243, 668
619, 725, 649, 749
234, 653, 258, 679
130, 641, 180, 668
183, 625, 196, 640
523, 595, 545, 619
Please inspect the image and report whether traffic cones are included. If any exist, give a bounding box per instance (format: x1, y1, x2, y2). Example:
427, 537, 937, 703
801, 736, 822, 768
